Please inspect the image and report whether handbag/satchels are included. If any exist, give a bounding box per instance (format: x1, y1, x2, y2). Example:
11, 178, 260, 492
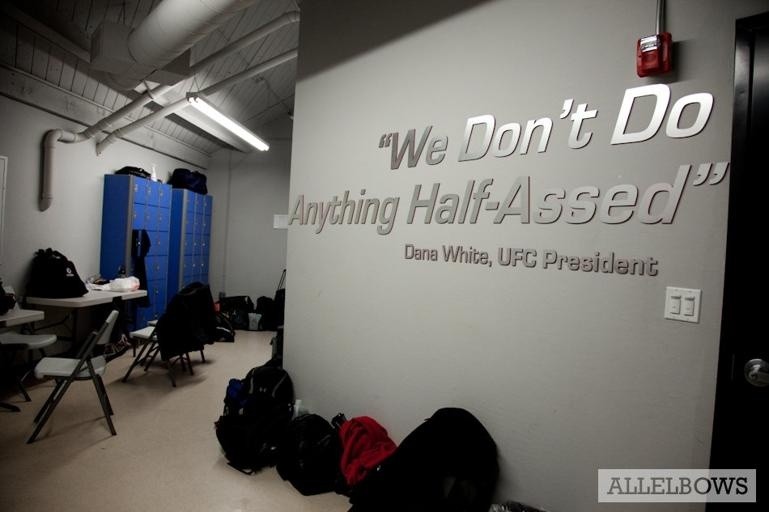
168, 168, 208, 194
29, 249, 89, 297
215, 292, 280, 342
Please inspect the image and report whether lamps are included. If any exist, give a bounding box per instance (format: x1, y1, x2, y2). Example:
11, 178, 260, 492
185, 74, 270, 151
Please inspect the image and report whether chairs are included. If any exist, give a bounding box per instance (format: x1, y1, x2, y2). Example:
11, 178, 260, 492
27, 309, 119, 446
122, 280, 213, 388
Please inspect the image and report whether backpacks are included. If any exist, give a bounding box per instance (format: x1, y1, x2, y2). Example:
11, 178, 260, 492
214, 361, 499, 512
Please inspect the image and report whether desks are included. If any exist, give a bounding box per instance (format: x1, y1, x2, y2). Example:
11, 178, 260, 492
0, 282, 148, 411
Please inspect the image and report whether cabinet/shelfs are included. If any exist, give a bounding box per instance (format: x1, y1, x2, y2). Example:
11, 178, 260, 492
99, 174, 214, 340
